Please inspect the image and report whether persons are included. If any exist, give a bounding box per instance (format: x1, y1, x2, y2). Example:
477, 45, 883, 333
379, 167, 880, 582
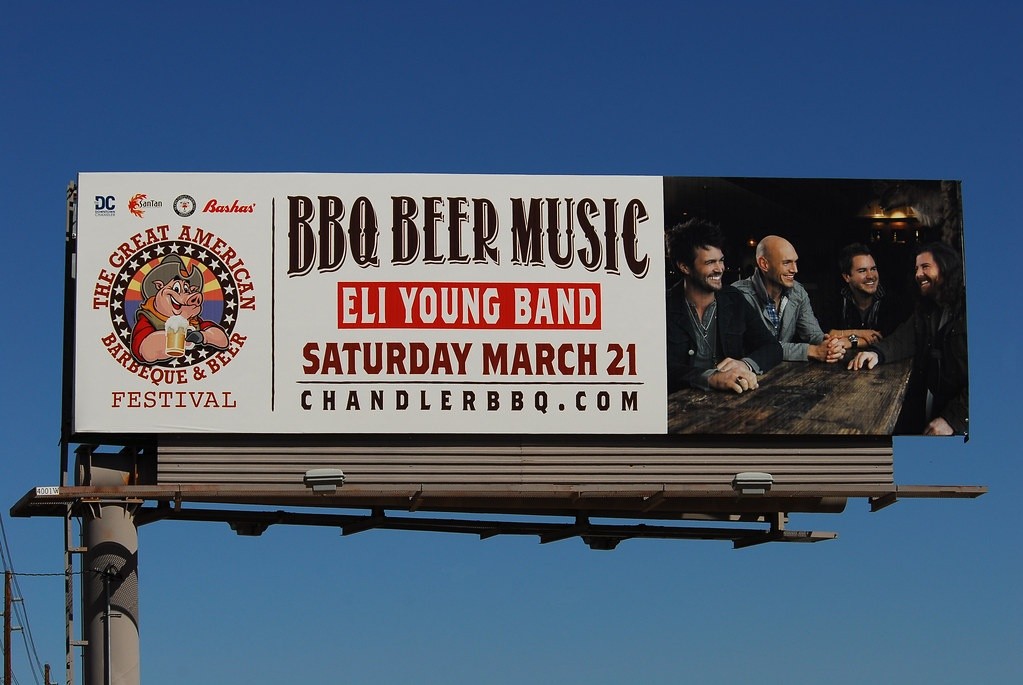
847, 241, 969, 436
666, 216, 783, 394
730, 235, 846, 363
816, 242, 897, 349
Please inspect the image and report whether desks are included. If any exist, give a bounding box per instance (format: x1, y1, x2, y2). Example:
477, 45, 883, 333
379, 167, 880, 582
668, 357, 911, 434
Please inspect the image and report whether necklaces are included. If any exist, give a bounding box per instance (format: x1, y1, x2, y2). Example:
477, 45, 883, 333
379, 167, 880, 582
685, 298, 716, 356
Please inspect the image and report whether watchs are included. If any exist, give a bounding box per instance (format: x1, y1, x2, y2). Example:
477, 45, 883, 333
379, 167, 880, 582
848, 334, 858, 349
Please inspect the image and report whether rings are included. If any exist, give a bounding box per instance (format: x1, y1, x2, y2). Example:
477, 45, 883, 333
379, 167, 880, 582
736, 375, 743, 383
